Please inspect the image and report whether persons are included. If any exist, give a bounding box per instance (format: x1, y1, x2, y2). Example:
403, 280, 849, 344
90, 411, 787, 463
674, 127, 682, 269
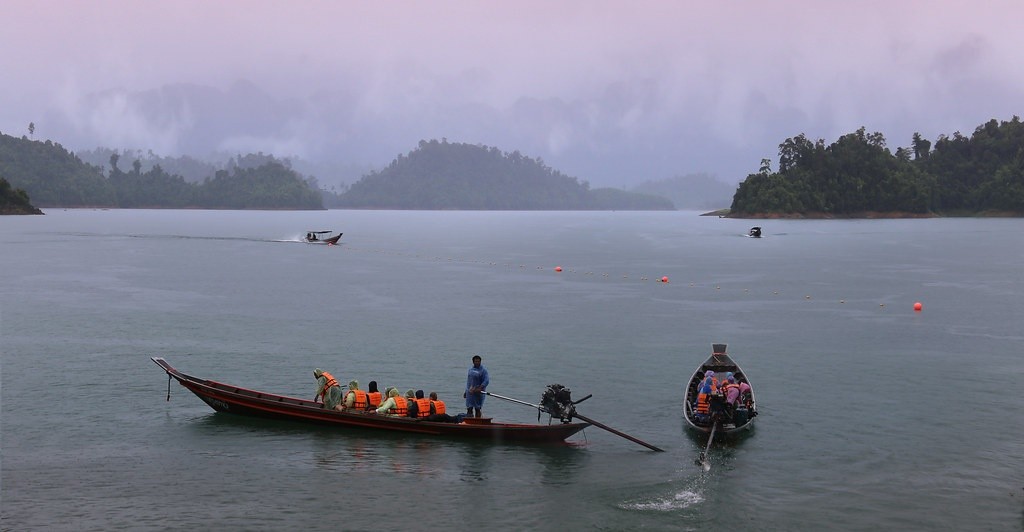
313, 234, 316, 239
697, 370, 750, 407
366, 381, 382, 411
370, 386, 407, 418
341, 381, 367, 412
406, 390, 430, 421
314, 368, 347, 411
429, 392, 445, 414
463, 356, 489, 418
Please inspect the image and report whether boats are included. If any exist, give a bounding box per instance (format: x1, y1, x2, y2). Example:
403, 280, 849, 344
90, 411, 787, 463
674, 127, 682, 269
682, 343, 757, 473
304, 229, 343, 245
152, 355, 666, 454
748, 226, 762, 236
